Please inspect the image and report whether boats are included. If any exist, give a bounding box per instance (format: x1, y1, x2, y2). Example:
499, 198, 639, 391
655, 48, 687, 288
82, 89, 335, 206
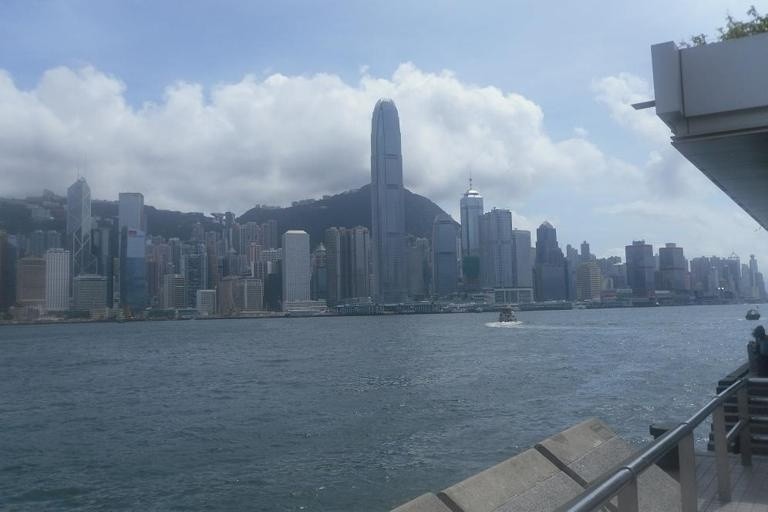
498, 306, 522, 326
